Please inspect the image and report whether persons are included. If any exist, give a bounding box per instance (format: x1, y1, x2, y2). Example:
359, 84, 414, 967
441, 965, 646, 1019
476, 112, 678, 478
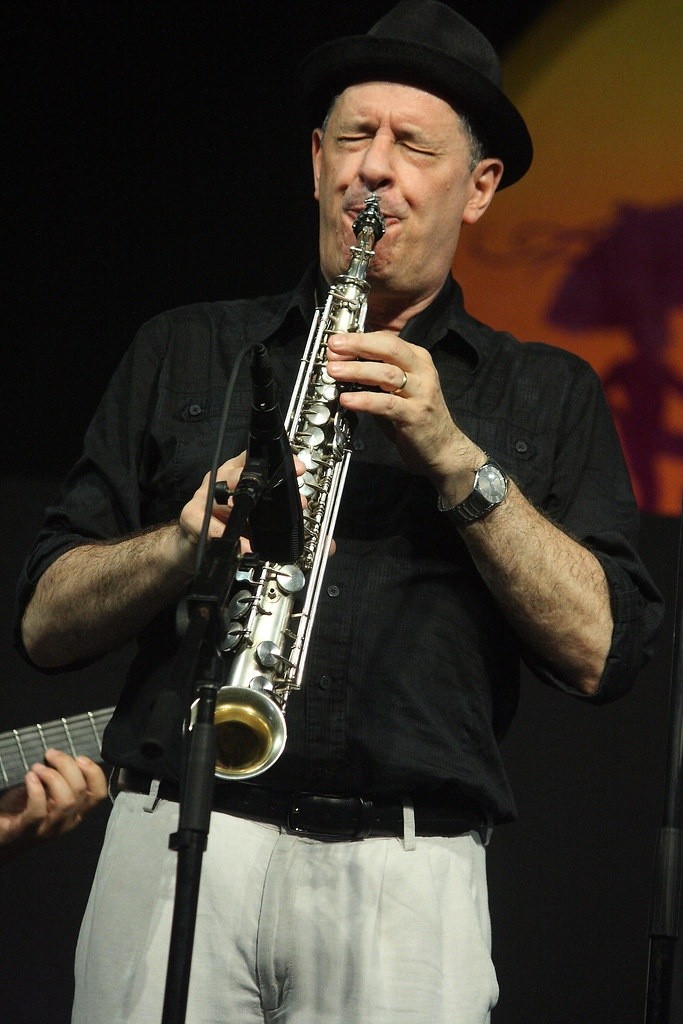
0, 746, 110, 850
8, 3, 669, 1024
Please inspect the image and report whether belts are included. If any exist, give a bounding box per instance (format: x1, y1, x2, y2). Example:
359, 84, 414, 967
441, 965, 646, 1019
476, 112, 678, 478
112, 763, 481, 840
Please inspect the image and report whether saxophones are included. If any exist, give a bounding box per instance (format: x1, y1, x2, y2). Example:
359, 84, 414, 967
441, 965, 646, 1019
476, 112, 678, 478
186, 193, 389, 783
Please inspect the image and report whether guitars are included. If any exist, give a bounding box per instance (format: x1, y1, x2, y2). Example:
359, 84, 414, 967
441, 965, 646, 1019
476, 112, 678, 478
1, 696, 128, 801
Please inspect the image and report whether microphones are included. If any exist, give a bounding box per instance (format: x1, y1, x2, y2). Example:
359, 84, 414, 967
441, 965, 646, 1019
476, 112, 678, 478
250, 342, 305, 564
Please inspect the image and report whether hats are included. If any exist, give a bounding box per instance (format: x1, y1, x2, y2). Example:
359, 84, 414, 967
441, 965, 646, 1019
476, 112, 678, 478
310, 0, 534, 193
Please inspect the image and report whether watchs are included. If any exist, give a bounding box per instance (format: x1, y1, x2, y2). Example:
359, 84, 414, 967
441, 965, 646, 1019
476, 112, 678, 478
437, 448, 510, 530
